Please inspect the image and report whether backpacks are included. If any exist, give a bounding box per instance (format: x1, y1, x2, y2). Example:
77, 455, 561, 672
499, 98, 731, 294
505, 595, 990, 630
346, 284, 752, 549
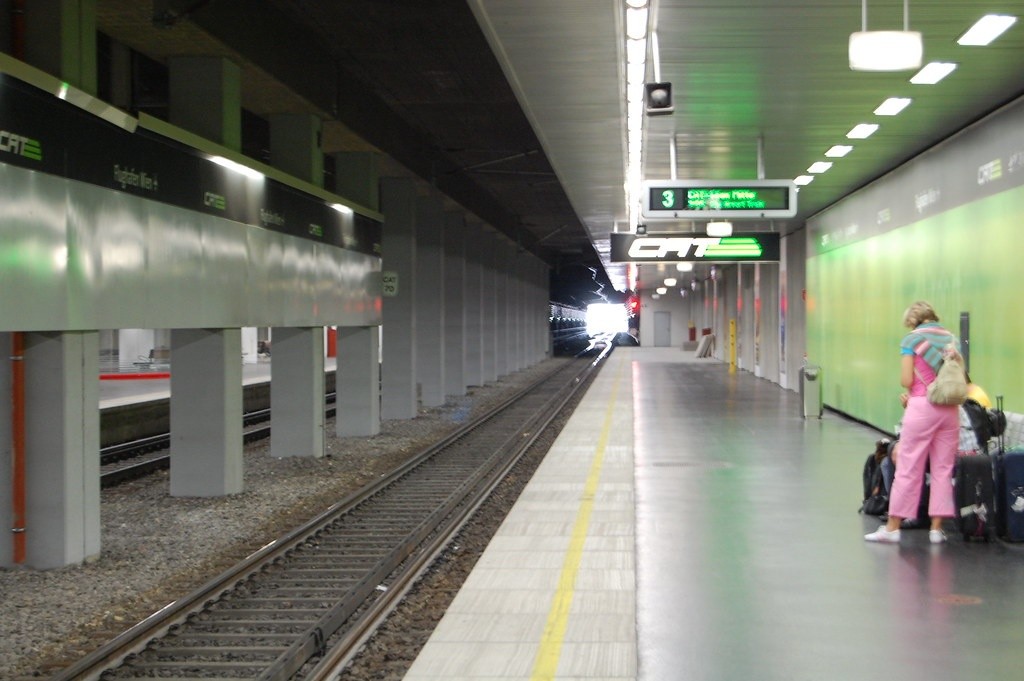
905, 331, 967, 405
958, 397, 1006, 448
862, 437, 897, 515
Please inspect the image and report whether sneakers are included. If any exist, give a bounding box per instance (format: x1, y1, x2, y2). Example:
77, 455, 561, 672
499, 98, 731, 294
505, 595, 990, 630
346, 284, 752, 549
864, 525, 901, 543
929, 529, 947, 543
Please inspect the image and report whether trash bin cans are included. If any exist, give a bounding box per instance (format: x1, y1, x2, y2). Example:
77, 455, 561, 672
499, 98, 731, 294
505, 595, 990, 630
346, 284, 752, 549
799, 365, 824, 420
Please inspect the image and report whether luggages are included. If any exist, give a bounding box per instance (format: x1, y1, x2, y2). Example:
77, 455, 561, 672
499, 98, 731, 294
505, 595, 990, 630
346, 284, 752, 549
954, 454, 998, 543
995, 396, 1024, 542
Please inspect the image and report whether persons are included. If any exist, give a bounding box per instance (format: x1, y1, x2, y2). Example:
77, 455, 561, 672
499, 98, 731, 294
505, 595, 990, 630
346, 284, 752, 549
863, 301, 989, 544
